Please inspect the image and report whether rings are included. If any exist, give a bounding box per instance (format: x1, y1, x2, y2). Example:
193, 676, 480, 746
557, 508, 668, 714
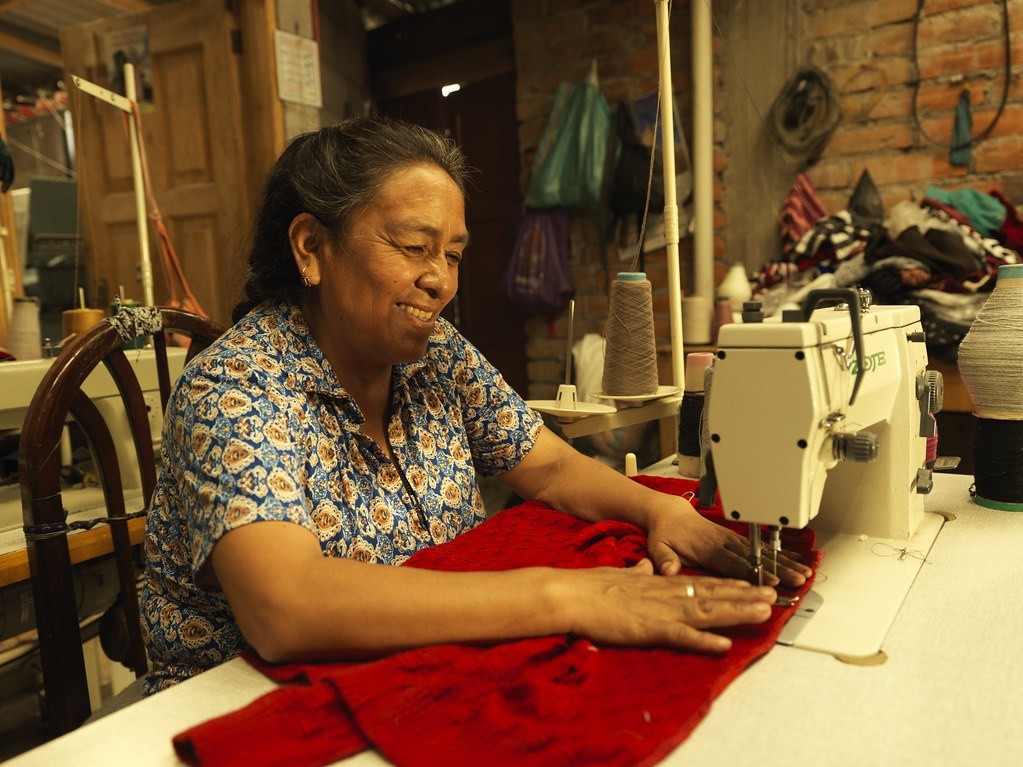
686, 583, 696, 598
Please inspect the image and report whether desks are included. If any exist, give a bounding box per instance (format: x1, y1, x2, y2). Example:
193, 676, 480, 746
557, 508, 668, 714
543, 397, 681, 461
0, 446, 1023, 764
0, 456, 148, 589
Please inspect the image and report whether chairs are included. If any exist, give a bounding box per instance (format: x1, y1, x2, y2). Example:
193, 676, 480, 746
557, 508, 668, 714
17, 306, 227, 744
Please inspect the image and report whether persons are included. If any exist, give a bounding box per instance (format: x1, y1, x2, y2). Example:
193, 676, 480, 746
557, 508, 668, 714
137, 116, 814, 701
